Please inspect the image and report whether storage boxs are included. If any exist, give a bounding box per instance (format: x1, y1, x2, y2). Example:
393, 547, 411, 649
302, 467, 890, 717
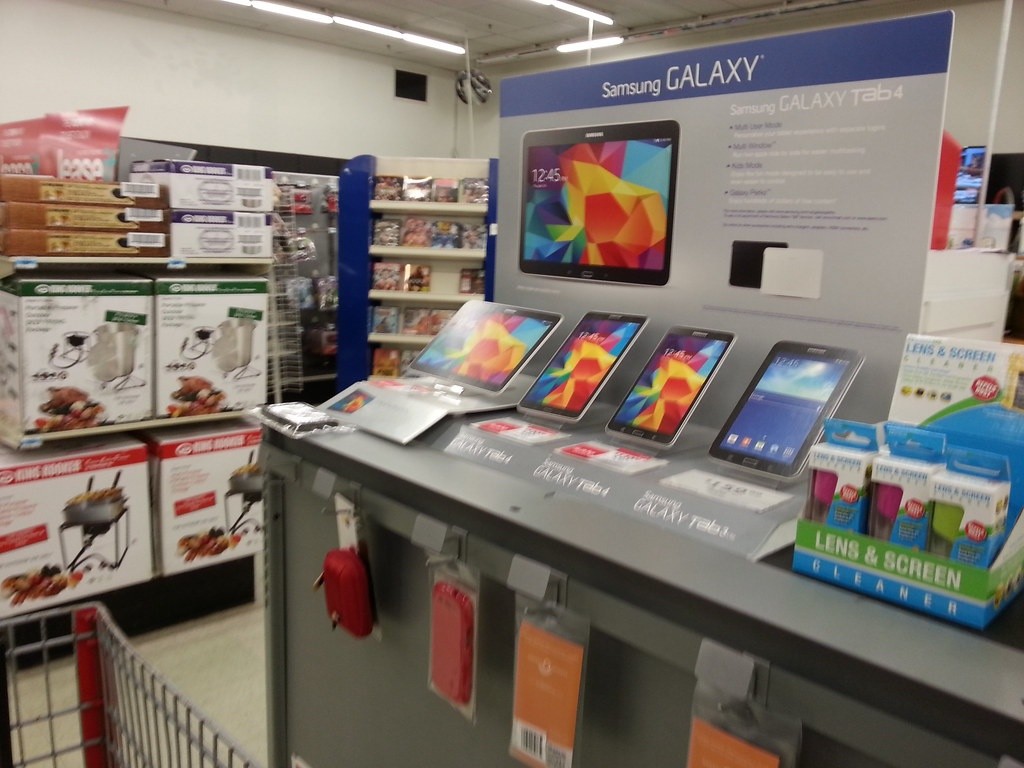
0, 202, 171, 234
0, 228, 171, 257
0, 272, 155, 436
132, 416, 263, 578
114, 268, 269, 419
0, 174, 171, 209
0, 433, 154, 621
167, 209, 273, 258
129, 158, 274, 212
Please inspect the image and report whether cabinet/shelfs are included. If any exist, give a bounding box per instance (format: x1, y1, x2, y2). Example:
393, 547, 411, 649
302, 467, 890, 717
0, 255, 274, 673
336, 154, 499, 395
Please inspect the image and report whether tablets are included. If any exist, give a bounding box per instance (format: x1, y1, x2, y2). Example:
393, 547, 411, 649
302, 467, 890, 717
605, 325, 737, 449
517, 310, 649, 423
708, 340, 866, 480
729, 240, 823, 298
409, 300, 565, 396
519, 119, 681, 285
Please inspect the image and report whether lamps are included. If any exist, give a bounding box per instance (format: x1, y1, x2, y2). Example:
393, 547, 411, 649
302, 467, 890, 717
530, 0, 614, 26
221, 0, 466, 55
556, 34, 624, 54
